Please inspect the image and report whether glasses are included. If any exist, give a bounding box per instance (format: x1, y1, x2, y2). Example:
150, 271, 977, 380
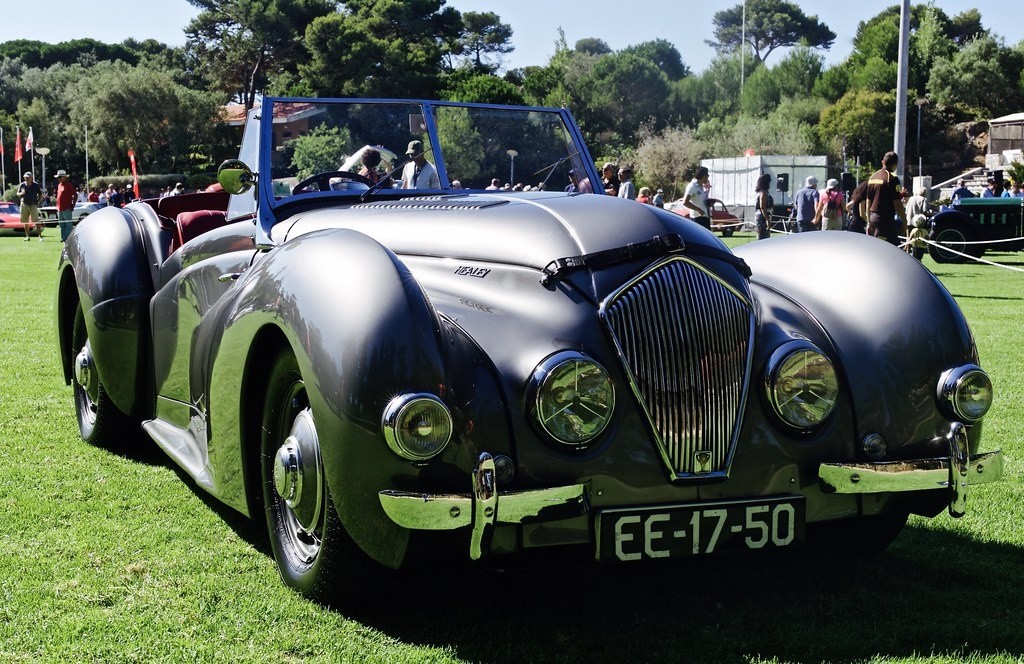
601, 167, 612, 172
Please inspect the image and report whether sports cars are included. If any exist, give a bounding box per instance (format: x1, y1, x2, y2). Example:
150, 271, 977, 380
55, 92, 1007, 616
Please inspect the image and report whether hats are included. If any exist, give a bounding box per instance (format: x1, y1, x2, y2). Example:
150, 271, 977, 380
569, 169, 578, 175
912, 214, 926, 228
1002, 181, 1012, 187
126, 184, 132, 188
825, 179, 839, 191
405, 140, 424, 155
54, 170, 70, 178
805, 176, 819, 188
23, 171, 33, 177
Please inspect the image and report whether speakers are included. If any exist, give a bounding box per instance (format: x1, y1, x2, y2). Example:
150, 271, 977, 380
777, 173, 789, 191
840, 172, 853, 191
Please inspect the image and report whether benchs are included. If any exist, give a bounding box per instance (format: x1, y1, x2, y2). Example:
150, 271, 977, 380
158, 192, 230, 229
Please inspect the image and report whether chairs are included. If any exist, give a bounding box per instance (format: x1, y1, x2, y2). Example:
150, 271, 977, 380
177, 210, 256, 246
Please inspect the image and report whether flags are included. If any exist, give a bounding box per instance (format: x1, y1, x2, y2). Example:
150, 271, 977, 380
26, 129, 33, 151
15, 127, 22, 162
0, 128, 5, 155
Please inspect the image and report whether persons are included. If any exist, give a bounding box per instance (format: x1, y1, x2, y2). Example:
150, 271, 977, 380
900, 187, 910, 210
16, 172, 44, 242
683, 167, 710, 232
794, 176, 867, 235
484, 178, 546, 192
866, 152, 907, 247
563, 162, 665, 209
908, 214, 930, 263
755, 174, 774, 240
353, 150, 380, 189
401, 140, 441, 189
906, 187, 930, 253
980, 180, 1024, 198
951, 178, 978, 204
46, 170, 186, 242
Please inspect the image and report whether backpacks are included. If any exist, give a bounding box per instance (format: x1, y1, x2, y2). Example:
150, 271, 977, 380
823, 192, 840, 219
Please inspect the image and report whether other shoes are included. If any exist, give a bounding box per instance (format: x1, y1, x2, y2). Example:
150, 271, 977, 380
24, 236, 30, 241
39, 237, 44, 242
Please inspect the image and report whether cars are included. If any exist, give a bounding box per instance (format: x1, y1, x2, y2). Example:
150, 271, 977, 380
0, 201, 46, 236
37, 201, 106, 229
